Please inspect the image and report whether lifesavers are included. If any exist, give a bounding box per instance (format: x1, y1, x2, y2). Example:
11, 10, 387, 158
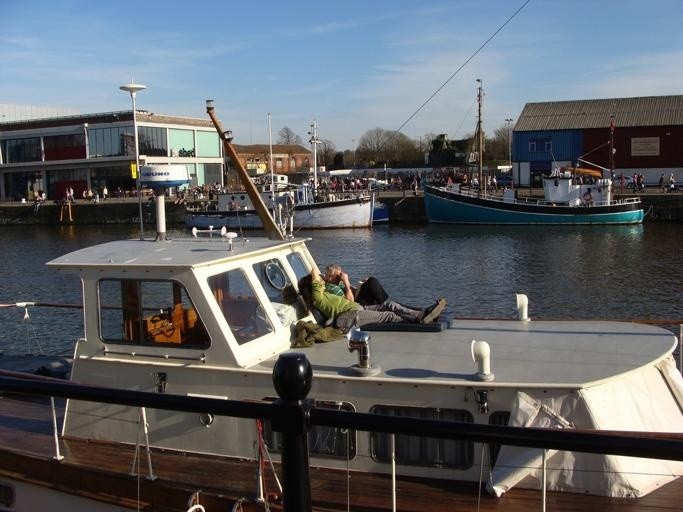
583, 192, 593, 201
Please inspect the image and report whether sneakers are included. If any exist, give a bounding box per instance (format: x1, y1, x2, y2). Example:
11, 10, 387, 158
421, 299, 445, 323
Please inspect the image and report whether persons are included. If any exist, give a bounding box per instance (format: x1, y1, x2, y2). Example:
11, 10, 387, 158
148, 191, 154, 207
251, 177, 272, 184
302, 266, 446, 330
632, 171, 676, 194
66, 187, 75, 199
265, 194, 273, 213
82, 185, 136, 203
331, 173, 422, 191
189, 182, 231, 194
302, 177, 335, 202
589, 176, 594, 185
578, 174, 583, 185
174, 191, 187, 204
432, 174, 497, 188
33, 191, 42, 211
582, 187, 594, 206
322, 263, 389, 307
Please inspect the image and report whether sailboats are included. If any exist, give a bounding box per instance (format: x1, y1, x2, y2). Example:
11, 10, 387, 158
423, 79, 645, 224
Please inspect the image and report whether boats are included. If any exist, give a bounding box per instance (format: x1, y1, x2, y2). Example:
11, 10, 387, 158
184, 111, 391, 228
0, 165, 683, 512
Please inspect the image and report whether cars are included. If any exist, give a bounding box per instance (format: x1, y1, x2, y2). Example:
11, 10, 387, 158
498, 176, 513, 186
614, 175, 634, 189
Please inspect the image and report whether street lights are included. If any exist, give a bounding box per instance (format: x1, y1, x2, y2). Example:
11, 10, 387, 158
505, 118, 513, 166
